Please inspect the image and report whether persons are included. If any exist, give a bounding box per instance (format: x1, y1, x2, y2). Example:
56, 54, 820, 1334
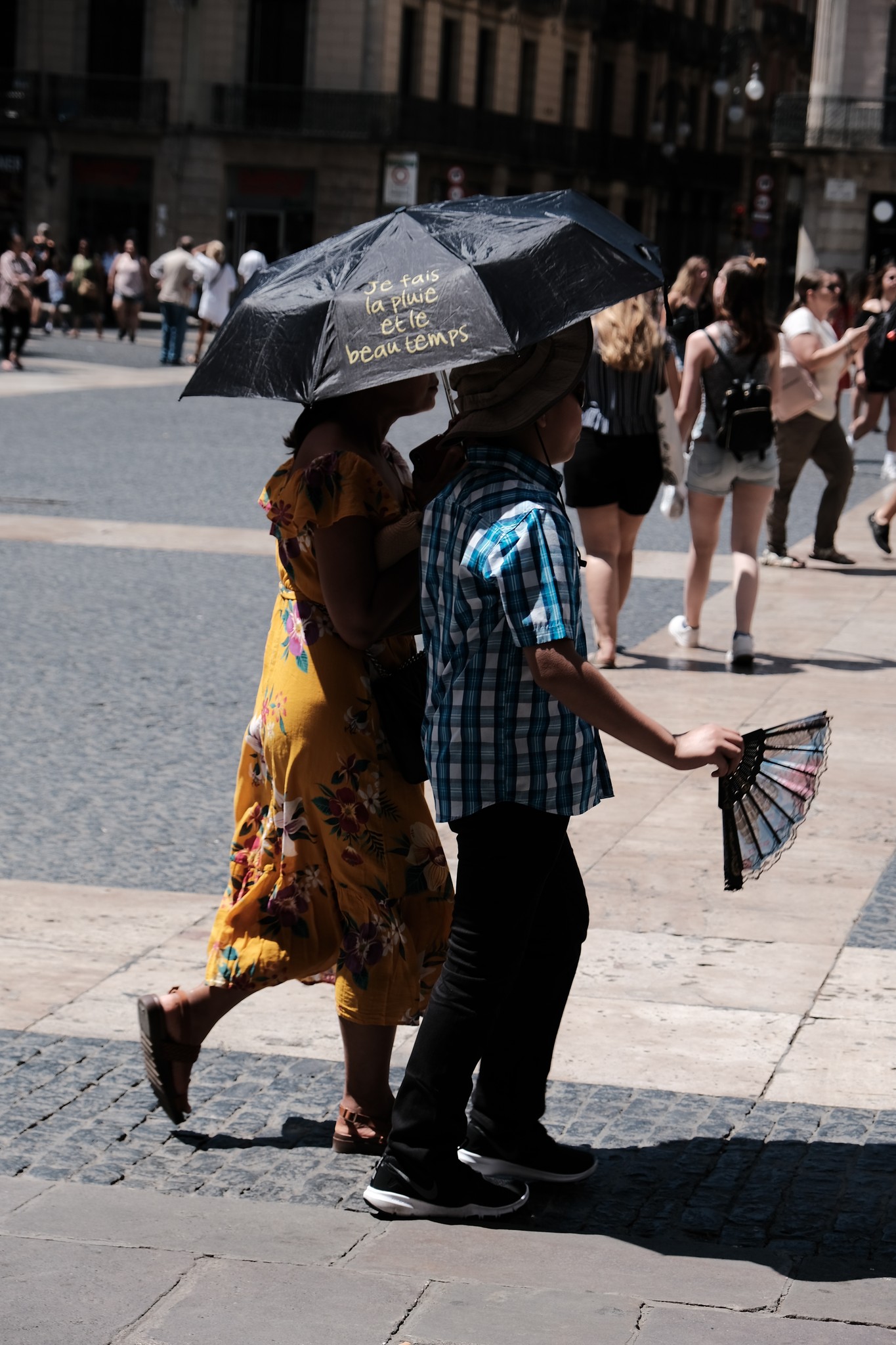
0, 222, 269, 372
139, 374, 454, 1158
359, 318, 746, 1219
561, 245, 896, 669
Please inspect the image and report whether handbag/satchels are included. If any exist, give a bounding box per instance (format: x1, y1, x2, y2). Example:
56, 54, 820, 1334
371, 650, 430, 785
654, 356, 685, 488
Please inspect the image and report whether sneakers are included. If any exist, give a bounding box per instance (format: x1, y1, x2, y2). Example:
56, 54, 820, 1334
363, 1152, 529, 1219
669, 614, 701, 647
730, 630, 755, 666
457, 1119, 599, 1182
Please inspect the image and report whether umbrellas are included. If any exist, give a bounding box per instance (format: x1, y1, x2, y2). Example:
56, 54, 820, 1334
179, 186, 675, 415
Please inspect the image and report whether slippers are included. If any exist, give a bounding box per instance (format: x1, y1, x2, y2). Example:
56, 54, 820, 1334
587, 618, 622, 669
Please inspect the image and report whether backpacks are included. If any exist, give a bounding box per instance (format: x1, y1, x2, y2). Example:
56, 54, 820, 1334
699, 327, 775, 463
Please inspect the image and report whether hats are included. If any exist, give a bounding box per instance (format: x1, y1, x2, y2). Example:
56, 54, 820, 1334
436, 314, 593, 449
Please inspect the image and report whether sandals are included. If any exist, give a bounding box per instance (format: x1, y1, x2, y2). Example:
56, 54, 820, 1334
808, 545, 856, 564
332, 1101, 468, 1156
759, 548, 805, 568
137, 986, 203, 1124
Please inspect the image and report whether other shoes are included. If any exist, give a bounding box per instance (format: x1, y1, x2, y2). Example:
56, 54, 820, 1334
845, 434, 858, 469
879, 452, 896, 480
8, 355, 23, 370
867, 512, 892, 554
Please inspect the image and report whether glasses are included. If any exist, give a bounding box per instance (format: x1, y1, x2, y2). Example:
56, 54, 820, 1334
816, 282, 840, 292
